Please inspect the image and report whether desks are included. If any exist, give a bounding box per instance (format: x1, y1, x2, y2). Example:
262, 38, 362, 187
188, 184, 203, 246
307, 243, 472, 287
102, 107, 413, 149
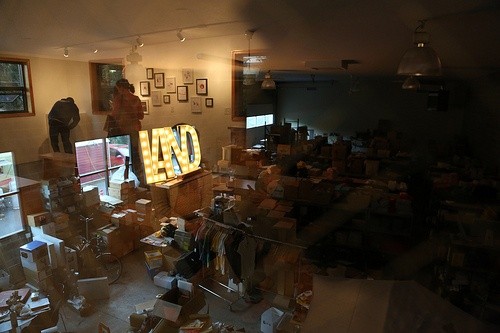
39, 152, 77, 178
0, 288, 45, 333
154, 169, 213, 221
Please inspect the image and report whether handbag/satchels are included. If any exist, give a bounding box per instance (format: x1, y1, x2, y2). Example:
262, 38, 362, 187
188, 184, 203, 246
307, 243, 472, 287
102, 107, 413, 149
102, 115, 116, 132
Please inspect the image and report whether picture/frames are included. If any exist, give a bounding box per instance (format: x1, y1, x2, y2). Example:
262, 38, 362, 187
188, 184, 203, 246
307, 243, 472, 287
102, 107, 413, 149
139, 68, 213, 115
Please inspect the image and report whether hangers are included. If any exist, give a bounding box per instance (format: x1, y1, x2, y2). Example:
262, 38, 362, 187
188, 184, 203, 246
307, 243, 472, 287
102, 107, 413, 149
195, 216, 303, 269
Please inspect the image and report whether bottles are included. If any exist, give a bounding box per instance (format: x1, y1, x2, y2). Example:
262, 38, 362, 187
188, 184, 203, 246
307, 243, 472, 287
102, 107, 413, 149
10, 309, 18, 328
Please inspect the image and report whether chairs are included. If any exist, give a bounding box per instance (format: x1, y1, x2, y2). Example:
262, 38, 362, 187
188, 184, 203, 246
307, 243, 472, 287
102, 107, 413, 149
20, 281, 68, 333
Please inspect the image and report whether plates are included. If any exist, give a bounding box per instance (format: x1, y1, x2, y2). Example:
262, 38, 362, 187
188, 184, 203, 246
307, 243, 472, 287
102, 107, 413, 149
0, 308, 9, 317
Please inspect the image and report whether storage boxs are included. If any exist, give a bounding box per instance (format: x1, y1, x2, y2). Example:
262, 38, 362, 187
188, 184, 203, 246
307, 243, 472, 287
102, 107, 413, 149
18, 180, 198, 333
222, 122, 352, 242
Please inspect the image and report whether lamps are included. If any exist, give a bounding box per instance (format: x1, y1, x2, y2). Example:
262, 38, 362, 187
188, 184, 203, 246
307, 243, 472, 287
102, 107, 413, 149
241, 30, 277, 90
397, 21, 443, 90
64, 31, 185, 57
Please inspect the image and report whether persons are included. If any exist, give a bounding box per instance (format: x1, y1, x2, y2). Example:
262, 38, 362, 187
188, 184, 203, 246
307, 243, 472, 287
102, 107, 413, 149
107, 79, 144, 176
387, 130, 401, 156
284, 153, 311, 177
48, 97, 80, 153
